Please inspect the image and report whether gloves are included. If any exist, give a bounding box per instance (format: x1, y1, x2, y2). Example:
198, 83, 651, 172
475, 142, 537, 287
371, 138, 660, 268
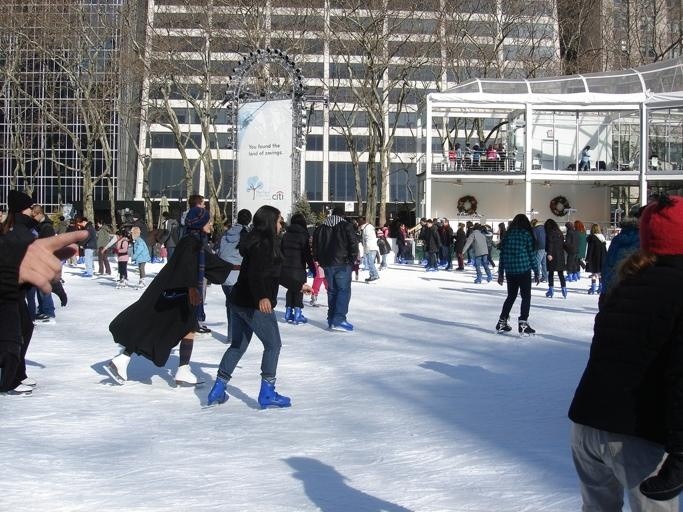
231, 265, 241, 271
639, 452, 683, 501
188, 287, 201, 307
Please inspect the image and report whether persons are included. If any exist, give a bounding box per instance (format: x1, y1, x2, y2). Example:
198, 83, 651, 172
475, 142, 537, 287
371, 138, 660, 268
648, 150, 658, 170
578, 146, 590, 170
449, 141, 517, 170
566, 195, 682, 511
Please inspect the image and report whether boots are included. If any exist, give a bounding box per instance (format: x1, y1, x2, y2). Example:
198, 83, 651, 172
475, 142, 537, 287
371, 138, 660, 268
68, 256, 161, 288
496, 318, 512, 331
293, 307, 308, 323
364, 257, 603, 297
285, 307, 294, 320
207, 376, 230, 404
518, 317, 536, 333
258, 376, 291, 406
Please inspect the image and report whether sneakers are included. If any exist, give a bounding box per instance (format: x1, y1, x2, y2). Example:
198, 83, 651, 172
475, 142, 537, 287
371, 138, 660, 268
328, 319, 354, 332
13, 383, 33, 392
175, 364, 206, 385
20, 377, 37, 386
109, 353, 131, 382
196, 325, 212, 333
35, 311, 56, 322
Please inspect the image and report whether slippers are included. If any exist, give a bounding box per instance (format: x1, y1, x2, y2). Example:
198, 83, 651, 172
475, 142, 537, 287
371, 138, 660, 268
310, 295, 320, 307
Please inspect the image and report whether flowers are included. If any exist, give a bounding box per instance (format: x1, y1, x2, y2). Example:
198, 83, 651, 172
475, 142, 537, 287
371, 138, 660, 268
456, 194, 477, 215
549, 196, 571, 217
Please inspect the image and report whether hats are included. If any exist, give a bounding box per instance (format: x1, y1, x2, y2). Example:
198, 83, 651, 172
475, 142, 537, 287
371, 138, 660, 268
130, 226, 140, 239
377, 229, 384, 237
184, 207, 210, 231
8, 189, 34, 212
639, 193, 683, 257
